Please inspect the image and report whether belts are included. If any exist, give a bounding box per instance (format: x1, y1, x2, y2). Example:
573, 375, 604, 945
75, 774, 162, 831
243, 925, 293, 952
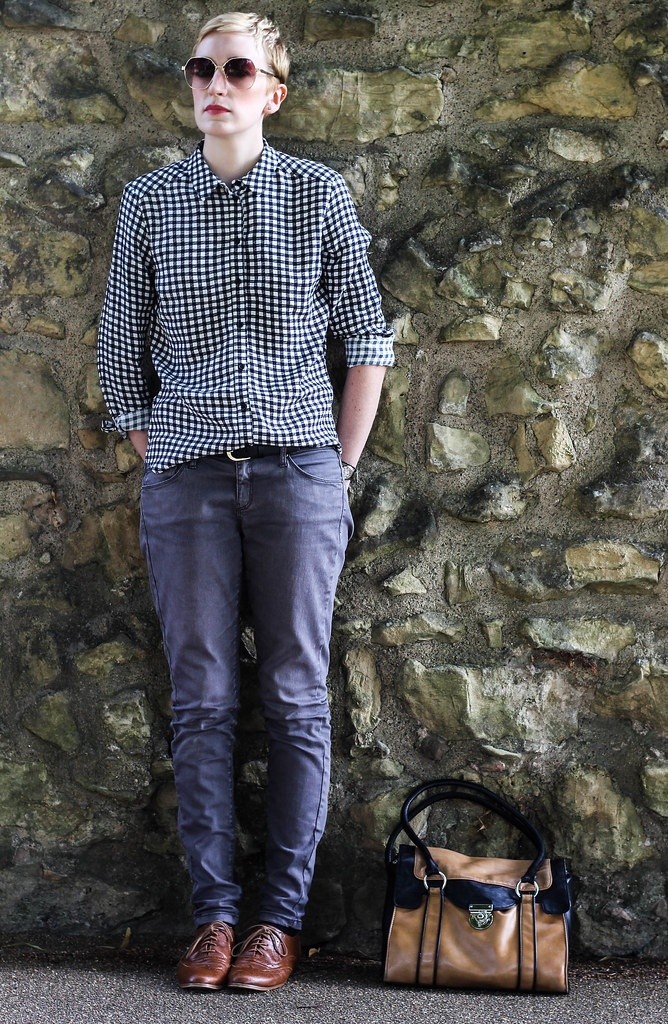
208, 445, 301, 460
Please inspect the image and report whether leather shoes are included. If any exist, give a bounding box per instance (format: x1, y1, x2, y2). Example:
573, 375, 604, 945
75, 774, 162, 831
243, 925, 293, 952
177, 920, 236, 988
226, 923, 301, 991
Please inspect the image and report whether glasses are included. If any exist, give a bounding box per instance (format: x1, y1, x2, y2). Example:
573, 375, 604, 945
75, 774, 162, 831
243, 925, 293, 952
181, 56, 285, 90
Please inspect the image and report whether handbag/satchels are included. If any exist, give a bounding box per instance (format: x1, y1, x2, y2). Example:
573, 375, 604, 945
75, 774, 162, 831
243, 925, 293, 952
383, 779, 573, 994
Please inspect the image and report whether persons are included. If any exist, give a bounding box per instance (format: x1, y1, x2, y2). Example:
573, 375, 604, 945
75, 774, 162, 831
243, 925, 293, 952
96, 11, 396, 990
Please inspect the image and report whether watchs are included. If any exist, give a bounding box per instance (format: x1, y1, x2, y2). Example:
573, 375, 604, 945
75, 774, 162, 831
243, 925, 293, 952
342, 461, 356, 480
